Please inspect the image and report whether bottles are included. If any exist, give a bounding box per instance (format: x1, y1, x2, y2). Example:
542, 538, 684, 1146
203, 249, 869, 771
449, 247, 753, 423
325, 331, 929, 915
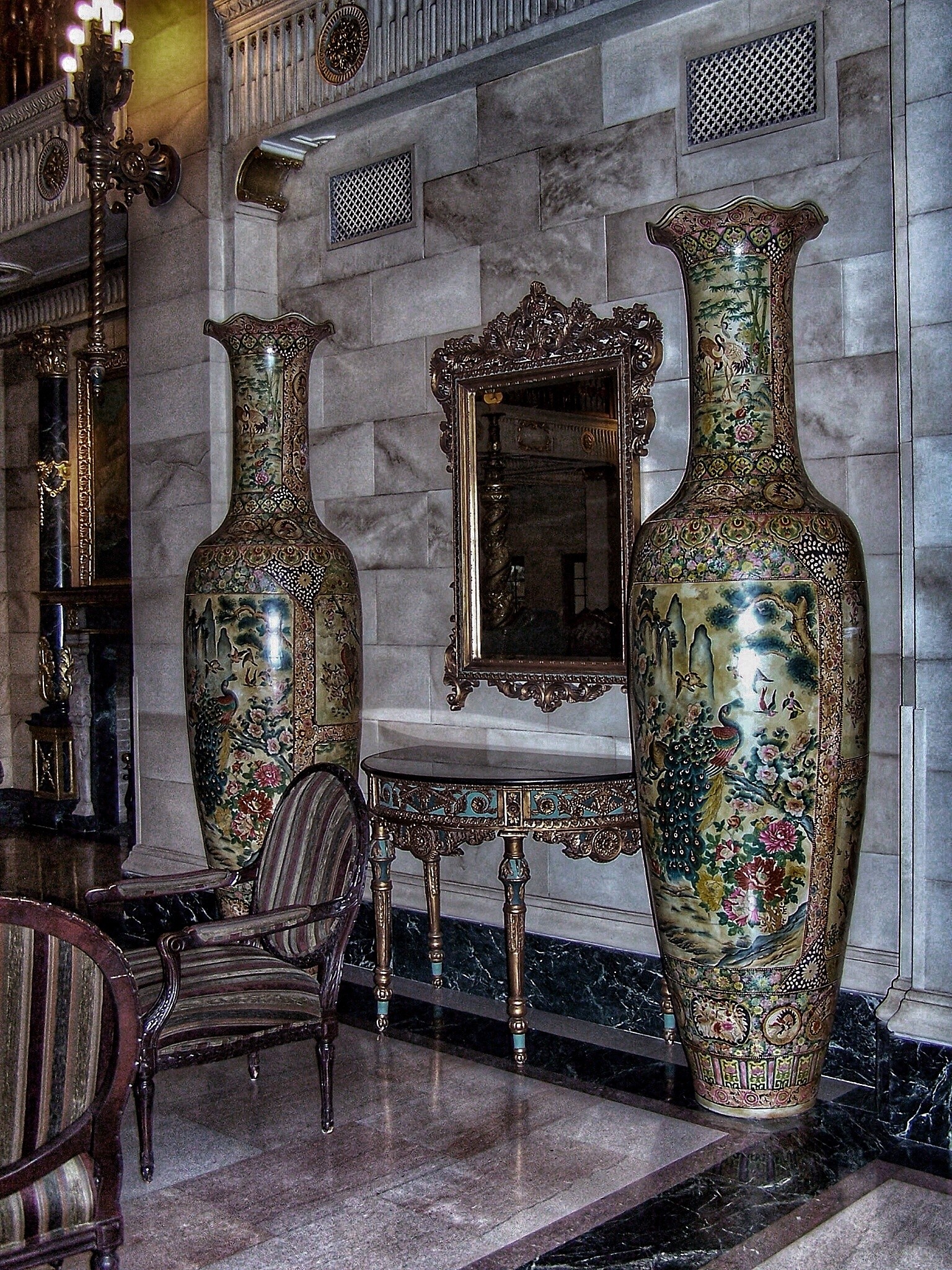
624, 196, 870, 1117
180, 313, 365, 920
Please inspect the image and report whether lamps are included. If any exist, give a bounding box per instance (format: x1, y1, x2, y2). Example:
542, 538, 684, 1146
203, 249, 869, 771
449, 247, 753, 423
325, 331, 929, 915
60, 0, 182, 208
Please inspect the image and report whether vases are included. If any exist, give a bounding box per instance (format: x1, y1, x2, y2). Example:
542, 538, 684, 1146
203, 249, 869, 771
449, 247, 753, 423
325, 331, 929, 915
627, 194, 871, 1119
183, 311, 364, 966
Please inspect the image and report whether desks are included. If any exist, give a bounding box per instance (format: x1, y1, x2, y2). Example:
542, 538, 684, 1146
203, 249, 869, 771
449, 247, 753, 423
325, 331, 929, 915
361, 745, 675, 1067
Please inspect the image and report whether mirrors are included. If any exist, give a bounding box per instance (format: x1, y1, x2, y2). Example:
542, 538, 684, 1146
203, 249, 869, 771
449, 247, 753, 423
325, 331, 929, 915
428, 281, 663, 713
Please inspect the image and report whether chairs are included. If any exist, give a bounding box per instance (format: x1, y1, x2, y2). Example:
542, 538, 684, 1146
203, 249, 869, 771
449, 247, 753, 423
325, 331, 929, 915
85, 762, 370, 1184
0, 896, 142, 1270
